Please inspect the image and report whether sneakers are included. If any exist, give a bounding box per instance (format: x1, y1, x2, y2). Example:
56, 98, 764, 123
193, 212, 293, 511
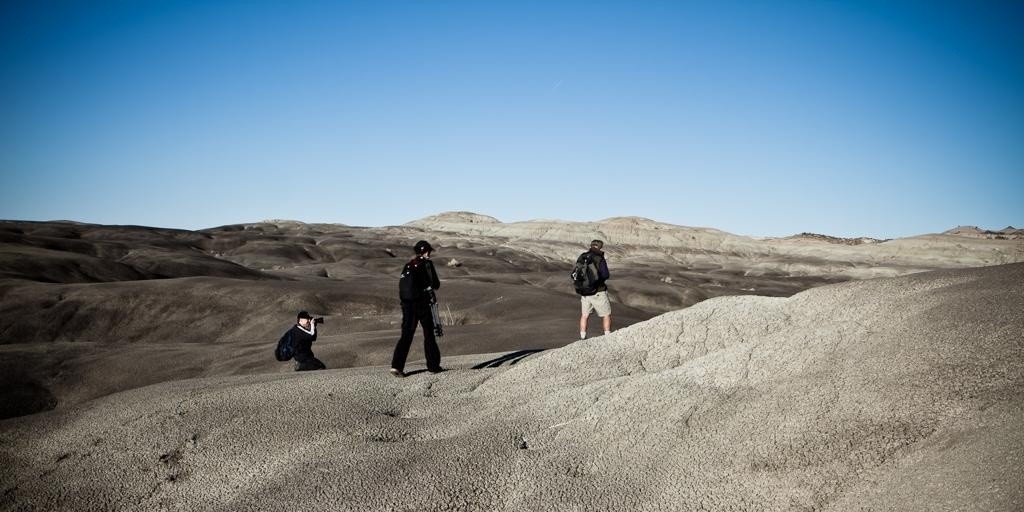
428, 367, 442, 375
390, 367, 405, 378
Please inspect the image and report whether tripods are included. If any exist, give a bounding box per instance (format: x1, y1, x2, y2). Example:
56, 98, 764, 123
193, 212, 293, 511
430, 290, 444, 336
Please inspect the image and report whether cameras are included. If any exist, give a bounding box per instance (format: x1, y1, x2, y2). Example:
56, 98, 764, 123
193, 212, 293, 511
310, 317, 323, 324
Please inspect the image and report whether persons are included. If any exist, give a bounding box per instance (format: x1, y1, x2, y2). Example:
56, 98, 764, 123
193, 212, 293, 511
391, 241, 443, 377
293, 311, 326, 371
576, 240, 612, 339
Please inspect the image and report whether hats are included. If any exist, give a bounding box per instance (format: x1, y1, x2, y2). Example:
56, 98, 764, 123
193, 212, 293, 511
297, 311, 313, 320
414, 240, 436, 253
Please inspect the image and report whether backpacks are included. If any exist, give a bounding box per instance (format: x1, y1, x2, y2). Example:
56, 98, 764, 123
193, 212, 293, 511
570, 252, 600, 296
398, 261, 424, 303
275, 328, 296, 361
